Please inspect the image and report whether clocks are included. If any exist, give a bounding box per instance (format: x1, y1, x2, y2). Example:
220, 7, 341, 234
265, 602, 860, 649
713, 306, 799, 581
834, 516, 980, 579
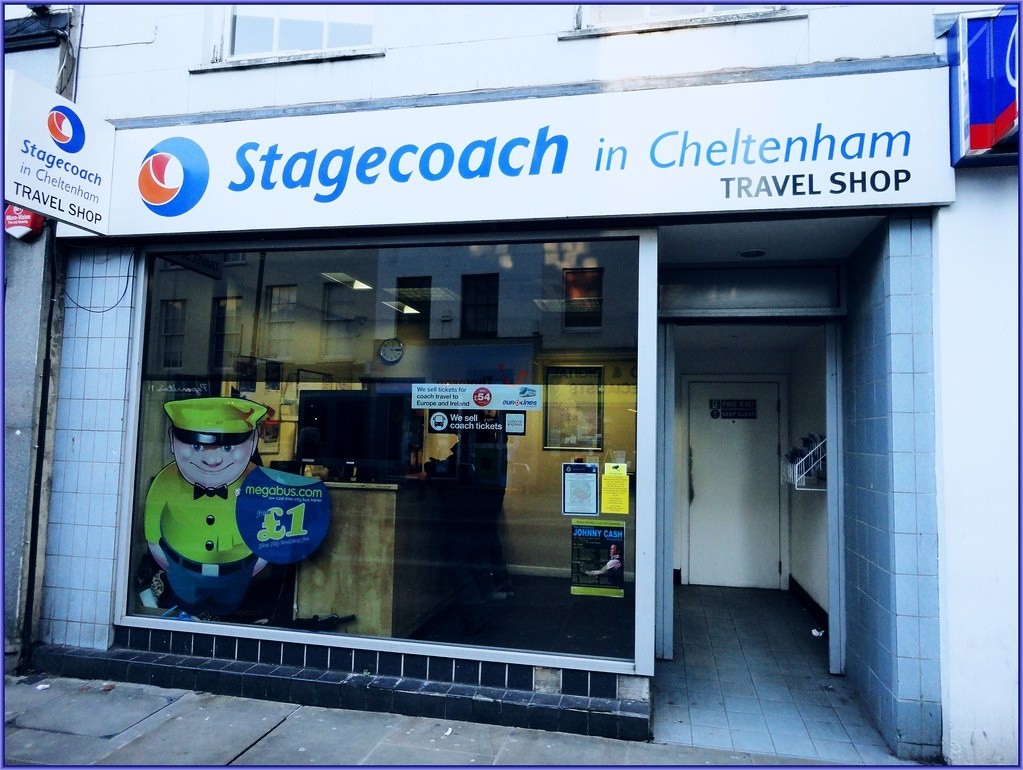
378, 338, 404, 364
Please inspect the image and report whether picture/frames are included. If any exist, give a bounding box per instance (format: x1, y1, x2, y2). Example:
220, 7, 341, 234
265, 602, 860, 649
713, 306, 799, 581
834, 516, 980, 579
563, 267, 606, 333
543, 365, 605, 452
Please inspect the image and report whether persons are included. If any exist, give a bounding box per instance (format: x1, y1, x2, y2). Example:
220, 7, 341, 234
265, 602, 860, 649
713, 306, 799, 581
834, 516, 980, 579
427, 410, 516, 633
585, 543, 623, 587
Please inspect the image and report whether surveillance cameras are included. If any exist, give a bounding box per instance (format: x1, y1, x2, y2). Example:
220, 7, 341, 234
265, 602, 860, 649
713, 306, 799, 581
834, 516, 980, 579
355, 317, 368, 327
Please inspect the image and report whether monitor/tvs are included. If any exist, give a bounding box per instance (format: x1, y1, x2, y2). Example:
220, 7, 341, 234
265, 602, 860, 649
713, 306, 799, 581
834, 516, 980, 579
296, 389, 424, 472
